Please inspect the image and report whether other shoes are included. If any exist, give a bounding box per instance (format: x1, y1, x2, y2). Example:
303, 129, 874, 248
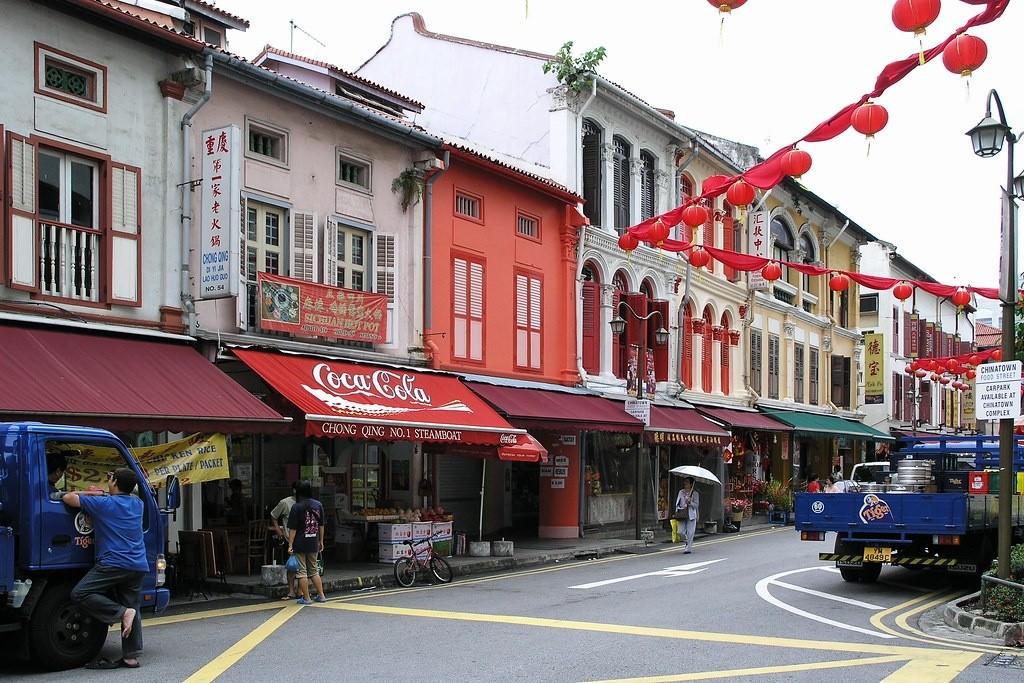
686, 542, 688, 546
684, 552, 691, 554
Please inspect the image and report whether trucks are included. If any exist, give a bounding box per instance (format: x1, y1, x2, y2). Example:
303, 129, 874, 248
0, 419, 181, 671
794, 433, 1024, 585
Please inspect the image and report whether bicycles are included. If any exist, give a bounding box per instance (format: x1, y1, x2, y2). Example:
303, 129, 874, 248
392, 530, 454, 587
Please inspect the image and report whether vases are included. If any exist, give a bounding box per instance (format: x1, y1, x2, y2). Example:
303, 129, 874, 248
732, 511, 743, 532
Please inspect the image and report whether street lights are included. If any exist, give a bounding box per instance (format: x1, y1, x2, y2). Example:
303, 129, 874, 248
966, 85, 1021, 601
606, 298, 670, 539
906, 383, 923, 433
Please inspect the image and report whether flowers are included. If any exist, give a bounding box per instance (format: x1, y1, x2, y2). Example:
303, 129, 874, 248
724, 496, 750, 513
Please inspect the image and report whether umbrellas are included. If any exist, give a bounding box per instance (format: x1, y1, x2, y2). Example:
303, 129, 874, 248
668, 463, 722, 497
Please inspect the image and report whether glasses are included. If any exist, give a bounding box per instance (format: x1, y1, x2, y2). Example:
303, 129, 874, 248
683, 481, 691, 483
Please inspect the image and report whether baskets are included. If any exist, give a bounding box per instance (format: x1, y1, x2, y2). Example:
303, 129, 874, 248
316, 552, 324, 576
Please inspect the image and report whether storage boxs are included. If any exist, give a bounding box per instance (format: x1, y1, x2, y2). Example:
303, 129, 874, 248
378, 522, 453, 564
767, 509, 790, 524
300, 464, 366, 559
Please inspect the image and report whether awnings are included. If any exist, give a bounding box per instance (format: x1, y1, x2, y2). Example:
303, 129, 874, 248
0, 323, 954, 444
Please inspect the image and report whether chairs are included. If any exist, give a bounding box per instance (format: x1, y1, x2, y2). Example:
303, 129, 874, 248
234, 519, 271, 576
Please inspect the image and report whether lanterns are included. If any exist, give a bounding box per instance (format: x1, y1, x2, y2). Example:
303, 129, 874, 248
852, 101, 888, 137
781, 148, 812, 181
648, 220, 670, 246
682, 205, 709, 232
689, 248, 1001, 392
727, 179, 755, 211
892, 0, 941, 36
619, 232, 638, 251
942, 33, 987, 76
708, 0, 748, 12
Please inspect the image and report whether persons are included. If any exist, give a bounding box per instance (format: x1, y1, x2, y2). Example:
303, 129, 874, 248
287, 481, 326, 604
808, 472, 820, 492
824, 476, 838, 493
62, 468, 149, 669
270, 481, 311, 599
46, 451, 67, 493
673, 477, 699, 553
831, 464, 843, 482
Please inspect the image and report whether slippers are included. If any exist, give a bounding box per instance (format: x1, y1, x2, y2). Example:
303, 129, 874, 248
85, 657, 119, 669
114, 658, 140, 668
281, 593, 304, 600
311, 595, 326, 603
297, 596, 311, 604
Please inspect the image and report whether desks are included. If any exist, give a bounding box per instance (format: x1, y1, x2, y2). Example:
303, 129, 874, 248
175, 529, 234, 600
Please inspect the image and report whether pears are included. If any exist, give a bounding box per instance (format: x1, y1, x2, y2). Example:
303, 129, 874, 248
363, 508, 395, 516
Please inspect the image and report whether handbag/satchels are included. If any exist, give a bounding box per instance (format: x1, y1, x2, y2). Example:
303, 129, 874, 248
675, 508, 689, 521
670, 519, 680, 543
285, 555, 301, 572
